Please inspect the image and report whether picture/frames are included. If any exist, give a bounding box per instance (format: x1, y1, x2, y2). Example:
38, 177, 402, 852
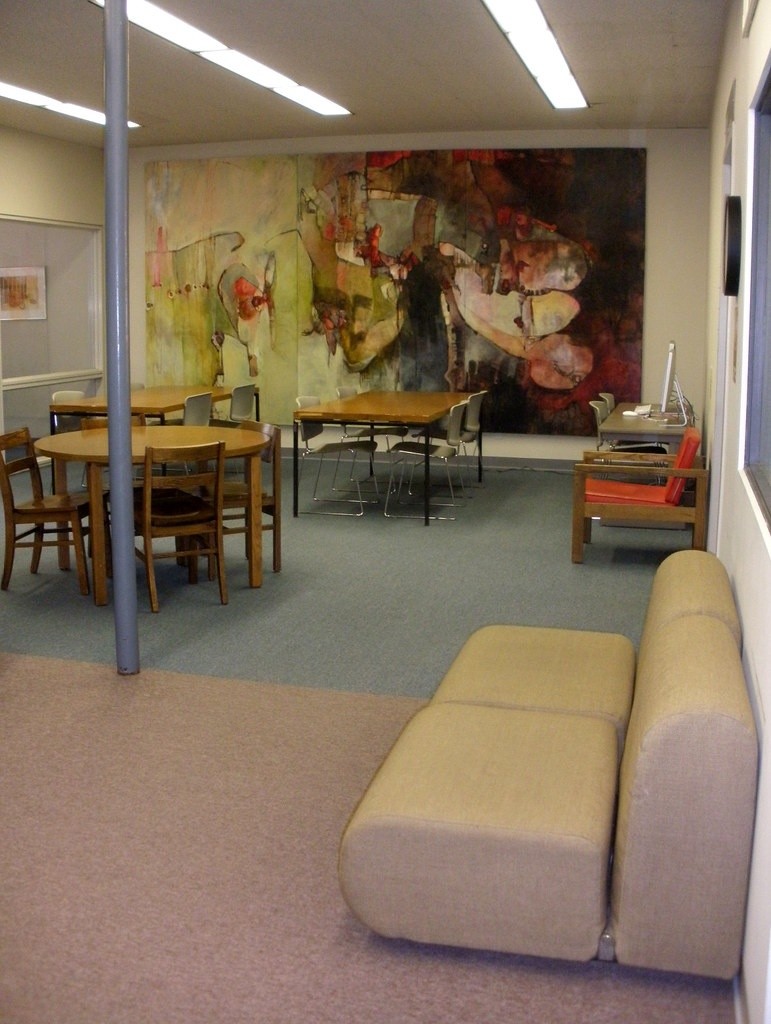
1, 266, 46, 320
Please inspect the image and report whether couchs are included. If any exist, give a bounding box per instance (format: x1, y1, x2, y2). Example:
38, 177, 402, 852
336, 541, 759, 984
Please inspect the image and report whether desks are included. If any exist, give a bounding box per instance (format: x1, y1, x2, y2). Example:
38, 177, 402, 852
600, 399, 697, 456
36, 423, 271, 591
49, 382, 259, 495
296, 388, 476, 528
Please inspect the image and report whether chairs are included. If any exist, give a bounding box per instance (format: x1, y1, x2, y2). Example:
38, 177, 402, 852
0, 368, 489, 613
589, 391, 669, 456
570, 425, 709, 567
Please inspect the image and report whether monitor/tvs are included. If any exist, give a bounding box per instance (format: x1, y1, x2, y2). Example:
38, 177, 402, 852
657, 340, 688, 426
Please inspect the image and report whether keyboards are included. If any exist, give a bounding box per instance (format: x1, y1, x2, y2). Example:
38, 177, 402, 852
634, 404, 652, 415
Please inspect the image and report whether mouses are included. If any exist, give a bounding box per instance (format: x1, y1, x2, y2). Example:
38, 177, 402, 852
623, 411, 638, 417
